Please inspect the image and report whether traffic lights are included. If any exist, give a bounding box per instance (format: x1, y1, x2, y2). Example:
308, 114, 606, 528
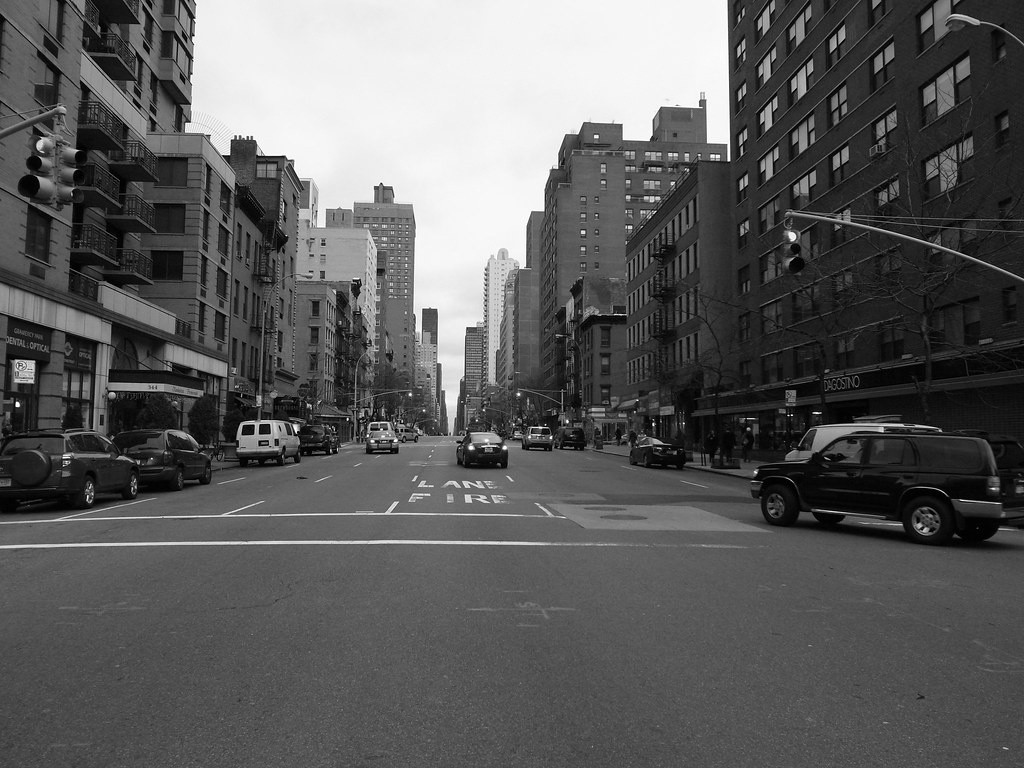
55, 138, 88, 204
409, 393, 412, 400
516, 392, 520, 401
17, 135, 59, 204
783, 230, 805, 275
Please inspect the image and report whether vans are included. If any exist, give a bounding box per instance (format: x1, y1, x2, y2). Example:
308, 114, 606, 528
236, 420, 303, 467
785, 423, 941, 469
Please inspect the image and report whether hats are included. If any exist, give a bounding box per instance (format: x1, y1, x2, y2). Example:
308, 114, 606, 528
747, 427, 751, 431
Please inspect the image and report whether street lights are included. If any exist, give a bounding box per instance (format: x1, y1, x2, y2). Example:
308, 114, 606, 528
354, 349, 379, 441
257, 273, 314, 417
555, 334, 585, 430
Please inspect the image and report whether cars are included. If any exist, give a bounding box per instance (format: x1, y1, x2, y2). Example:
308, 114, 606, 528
298, 421, 419, 457
106, 427, 214, 491
455, 432, 509, 468
512, 431, 524, 441
629, 437, 687, 470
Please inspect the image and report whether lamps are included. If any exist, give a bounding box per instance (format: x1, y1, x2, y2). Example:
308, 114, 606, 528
103, 391, 116, 401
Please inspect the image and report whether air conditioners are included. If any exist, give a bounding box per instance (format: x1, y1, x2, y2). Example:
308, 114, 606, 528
869, 144, 884, 156
230, 367, 238, 375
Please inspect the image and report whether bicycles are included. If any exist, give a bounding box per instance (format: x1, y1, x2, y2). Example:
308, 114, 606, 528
209, 440, 225, 461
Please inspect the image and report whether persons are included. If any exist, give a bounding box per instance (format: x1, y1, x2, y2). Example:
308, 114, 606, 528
674, 427, 684, 443
628, 430, 637, 447
592, 427, 599, 448
638, 430, 646, 440
434, 431, 451, 436
741, 427, 755, 462
708, 430, 719, 462
615, 427, 621, 445
723, 428, 737, 462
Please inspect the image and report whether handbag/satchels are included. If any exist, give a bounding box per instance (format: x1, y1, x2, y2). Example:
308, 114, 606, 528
628, 441, 631, 448
743, 439, 749, 446
635, 440, 638, 444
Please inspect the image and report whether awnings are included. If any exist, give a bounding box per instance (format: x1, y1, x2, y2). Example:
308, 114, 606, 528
618, 399, 637, 410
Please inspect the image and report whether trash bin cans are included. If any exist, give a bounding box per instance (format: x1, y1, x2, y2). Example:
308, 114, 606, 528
595, 435, 603, 450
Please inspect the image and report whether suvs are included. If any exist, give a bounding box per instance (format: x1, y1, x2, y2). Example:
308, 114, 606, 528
749, 429, 1024, 545
521, 427, 555, 451
553, 427, 587, 450
1, 430, 145, 509
